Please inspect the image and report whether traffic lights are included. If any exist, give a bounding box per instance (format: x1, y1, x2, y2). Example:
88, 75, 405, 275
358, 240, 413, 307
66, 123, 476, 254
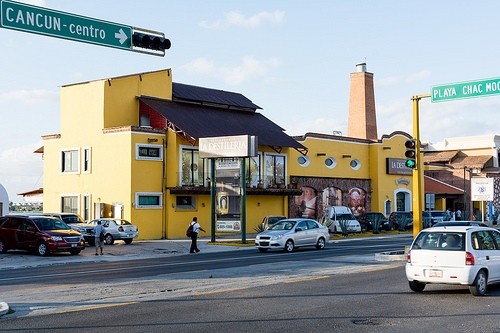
405, 139, 417, 170
134, 32, 170, 51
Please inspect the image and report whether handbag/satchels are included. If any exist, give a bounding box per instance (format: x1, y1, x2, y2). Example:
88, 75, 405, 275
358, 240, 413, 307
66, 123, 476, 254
100, 232, 105, 242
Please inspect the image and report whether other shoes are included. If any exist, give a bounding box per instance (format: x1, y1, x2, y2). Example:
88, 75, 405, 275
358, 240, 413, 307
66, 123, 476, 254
95, 253, 99, 255
196, 250, 200, 253
100, 253, 104, 255
190, 251, 195, 253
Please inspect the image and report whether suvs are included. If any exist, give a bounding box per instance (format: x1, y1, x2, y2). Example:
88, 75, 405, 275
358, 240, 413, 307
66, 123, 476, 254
391, 211, 414, 230
421, 213, 433, 228
53, 213, 96, 246
427, 210, 446, 224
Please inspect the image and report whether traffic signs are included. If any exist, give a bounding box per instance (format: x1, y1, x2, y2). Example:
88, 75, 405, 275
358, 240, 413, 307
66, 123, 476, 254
0, 0, 133, 52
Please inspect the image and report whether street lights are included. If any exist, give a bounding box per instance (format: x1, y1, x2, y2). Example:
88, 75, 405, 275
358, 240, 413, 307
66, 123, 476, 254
463, 166, 471, 220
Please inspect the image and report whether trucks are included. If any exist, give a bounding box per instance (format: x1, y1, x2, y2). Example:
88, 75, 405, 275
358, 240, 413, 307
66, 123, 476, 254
327, 206, 362, 233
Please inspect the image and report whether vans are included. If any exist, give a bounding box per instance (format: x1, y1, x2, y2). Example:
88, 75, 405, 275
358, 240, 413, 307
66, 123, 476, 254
0, 216, 85, 256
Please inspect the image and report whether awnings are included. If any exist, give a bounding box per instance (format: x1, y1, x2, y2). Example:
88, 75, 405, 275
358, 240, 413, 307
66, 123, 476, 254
17, 187, 43, 197
33, 146, 44, 154
135, 94, 308, 156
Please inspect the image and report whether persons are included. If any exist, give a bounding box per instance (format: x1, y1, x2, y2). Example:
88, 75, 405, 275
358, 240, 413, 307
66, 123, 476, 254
93, 221, 106, 255
445, 207, 500, 221
189, 217, 207, 253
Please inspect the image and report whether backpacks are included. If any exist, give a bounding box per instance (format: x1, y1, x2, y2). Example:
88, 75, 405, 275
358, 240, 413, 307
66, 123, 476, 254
186, 221, 197, 237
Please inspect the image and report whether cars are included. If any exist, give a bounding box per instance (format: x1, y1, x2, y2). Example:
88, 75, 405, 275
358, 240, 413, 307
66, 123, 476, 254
363, 212, 389, 231
254, 218, 330, 252
405, 227, 500, 296
433, 221, 489, 227
261, 215, 287, 230
88, 219, 138, 244
